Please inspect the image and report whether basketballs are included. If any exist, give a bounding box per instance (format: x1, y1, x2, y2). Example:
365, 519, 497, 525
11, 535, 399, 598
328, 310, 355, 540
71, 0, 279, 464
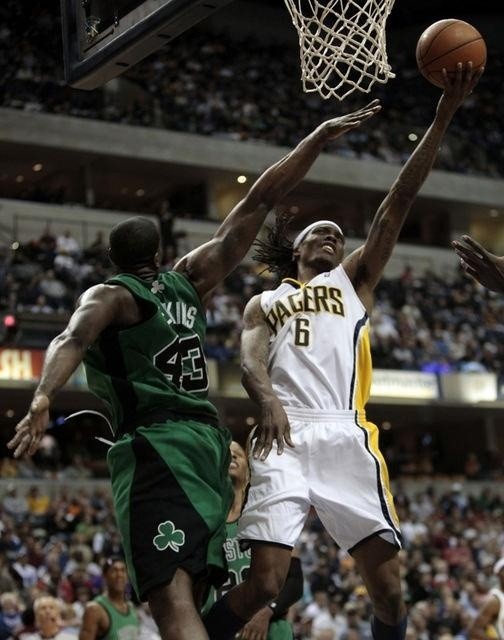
417, 19, 487, 88
